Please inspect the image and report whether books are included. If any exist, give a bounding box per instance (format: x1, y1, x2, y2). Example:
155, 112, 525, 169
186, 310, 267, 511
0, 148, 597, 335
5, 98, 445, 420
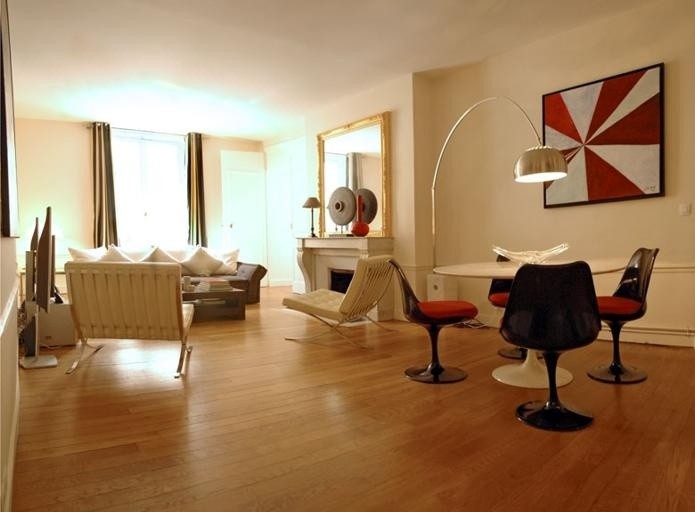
200, 277, 233, 291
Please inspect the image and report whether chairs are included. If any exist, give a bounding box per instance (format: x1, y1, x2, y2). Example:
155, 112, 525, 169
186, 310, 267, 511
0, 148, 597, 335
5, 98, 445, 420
578, 243, 658, 386
282, 252, 395, 355
59, 256, 197, 382
496, 258, 602, 433
380, 255, 480, 385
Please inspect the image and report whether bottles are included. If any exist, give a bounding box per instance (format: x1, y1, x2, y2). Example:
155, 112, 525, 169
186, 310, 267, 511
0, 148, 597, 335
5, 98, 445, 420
351, 194, 369, 237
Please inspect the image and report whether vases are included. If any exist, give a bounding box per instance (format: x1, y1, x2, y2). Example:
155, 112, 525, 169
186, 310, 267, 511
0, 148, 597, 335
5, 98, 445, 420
349, 194, 371, 236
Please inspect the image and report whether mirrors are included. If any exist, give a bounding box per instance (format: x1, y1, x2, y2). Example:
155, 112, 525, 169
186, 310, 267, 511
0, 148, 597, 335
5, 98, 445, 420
318, 110, 394, 238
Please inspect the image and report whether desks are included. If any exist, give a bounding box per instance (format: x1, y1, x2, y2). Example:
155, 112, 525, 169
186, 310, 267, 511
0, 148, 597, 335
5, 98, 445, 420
431, 260, 629, 391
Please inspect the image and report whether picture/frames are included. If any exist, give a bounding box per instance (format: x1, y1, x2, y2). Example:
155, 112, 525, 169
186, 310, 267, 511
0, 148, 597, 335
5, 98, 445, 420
540, 62, 665, 208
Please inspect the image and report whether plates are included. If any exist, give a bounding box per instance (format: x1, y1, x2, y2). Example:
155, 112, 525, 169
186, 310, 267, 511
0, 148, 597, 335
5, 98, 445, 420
492, 241, 570, 267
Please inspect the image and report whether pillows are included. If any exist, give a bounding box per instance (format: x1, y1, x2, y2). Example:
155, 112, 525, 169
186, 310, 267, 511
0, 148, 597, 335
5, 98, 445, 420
135, 244, 182, 264
98, 243, 132, 263
180, 243, 222, 277
215, 247, 241, 272
68, 245, 106, 260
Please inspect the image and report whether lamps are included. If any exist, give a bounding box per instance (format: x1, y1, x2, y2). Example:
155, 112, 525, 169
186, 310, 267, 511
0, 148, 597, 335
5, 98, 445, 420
302, 194, 321, 236
429, 90, 569, 272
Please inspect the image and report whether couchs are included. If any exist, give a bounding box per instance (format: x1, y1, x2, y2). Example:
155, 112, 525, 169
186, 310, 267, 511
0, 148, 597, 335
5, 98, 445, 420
69, 241, 267, 318
